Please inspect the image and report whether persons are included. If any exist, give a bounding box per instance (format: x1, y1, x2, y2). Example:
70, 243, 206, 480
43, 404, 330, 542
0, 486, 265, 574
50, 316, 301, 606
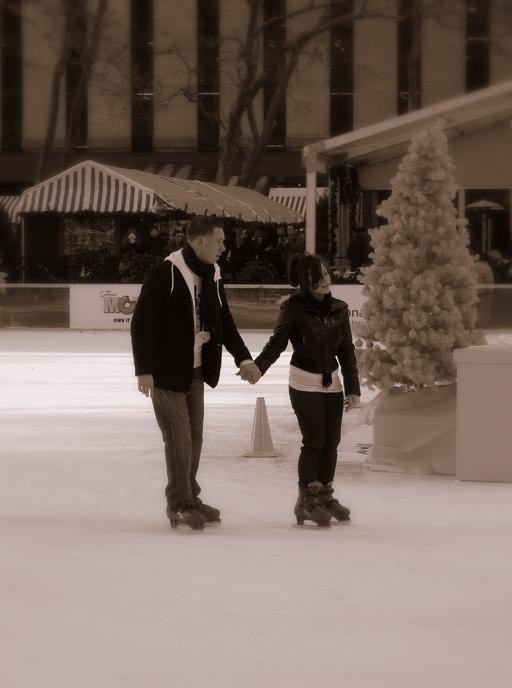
346, 227, 371, 270
130, 215, 260, 526
476, 253, 495, 284
235, 254, 361, 522
117, 224, 306, 283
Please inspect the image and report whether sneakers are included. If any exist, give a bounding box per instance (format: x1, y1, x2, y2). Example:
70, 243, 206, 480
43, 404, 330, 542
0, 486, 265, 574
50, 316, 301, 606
165, 504, 220, 525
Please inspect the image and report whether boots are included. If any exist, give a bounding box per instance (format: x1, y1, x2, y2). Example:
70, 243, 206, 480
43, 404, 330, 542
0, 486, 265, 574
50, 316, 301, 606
294, 482, 350, 520
